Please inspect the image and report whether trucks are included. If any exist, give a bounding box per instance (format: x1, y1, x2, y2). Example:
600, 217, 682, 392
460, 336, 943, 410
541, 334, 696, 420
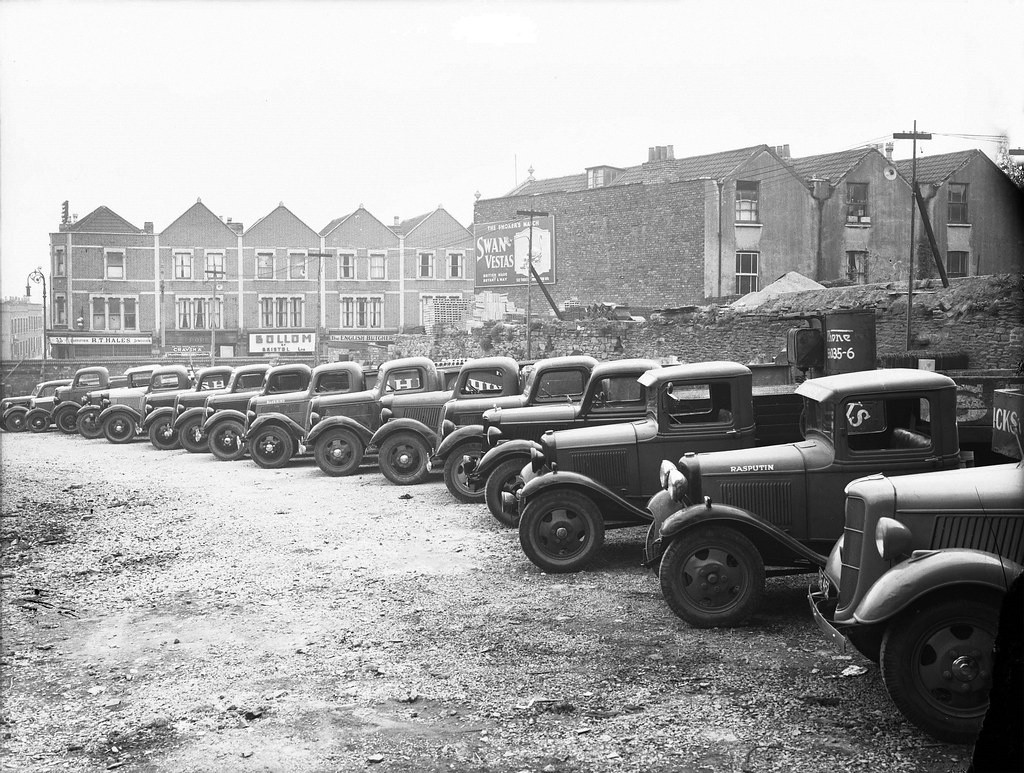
1, 352, 1024, 747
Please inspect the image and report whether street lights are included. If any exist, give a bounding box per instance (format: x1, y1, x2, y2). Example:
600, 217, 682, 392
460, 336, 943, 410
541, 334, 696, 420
25, 270, 46, 359
